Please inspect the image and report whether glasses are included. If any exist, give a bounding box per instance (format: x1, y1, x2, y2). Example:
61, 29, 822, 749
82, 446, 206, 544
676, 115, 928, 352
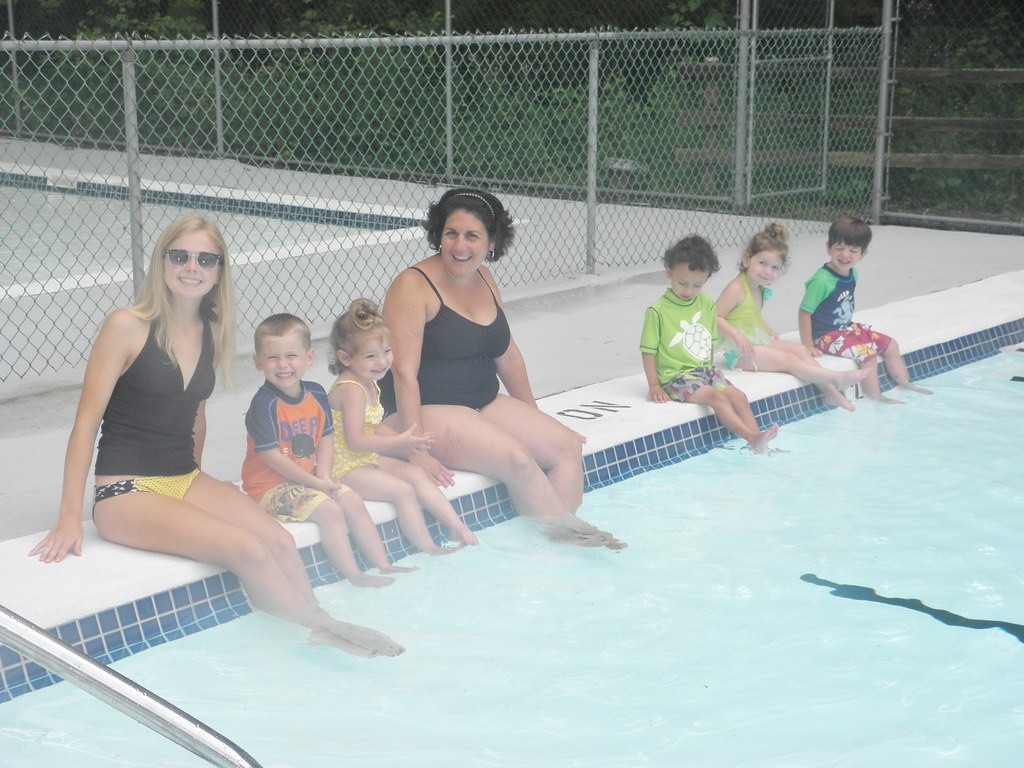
166, 249, 221, 271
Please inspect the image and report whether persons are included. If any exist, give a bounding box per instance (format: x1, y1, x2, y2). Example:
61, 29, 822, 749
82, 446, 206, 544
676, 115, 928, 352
377, 188, 586, 519
639, 234, 779, 450
715, 225, 871, 407
801, 217, 909, 397
326, 298, 480, 542
241, 314, 390, 575
28, 217, 318, 606
